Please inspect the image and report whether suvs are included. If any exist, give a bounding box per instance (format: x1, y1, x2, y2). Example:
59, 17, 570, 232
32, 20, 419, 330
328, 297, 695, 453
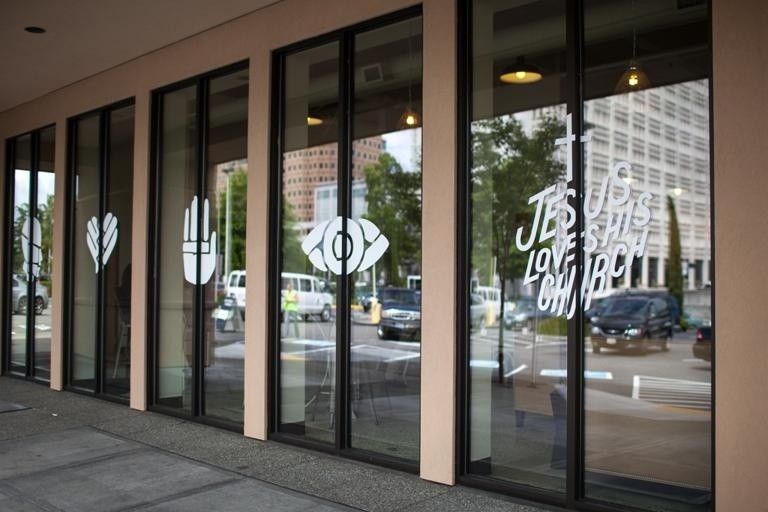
377, 287, 421, 342
590, 297, 675, 356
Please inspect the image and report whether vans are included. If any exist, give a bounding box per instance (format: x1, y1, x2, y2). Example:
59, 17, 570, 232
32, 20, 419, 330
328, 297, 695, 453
225, 268, 335, 322
585, 291, 680, 332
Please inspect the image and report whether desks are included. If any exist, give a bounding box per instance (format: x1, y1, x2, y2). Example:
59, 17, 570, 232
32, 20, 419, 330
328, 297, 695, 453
304, 343, 421, 426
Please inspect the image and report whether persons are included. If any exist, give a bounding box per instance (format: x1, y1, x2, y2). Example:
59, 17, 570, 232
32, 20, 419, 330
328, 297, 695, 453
284, 281, 301, 339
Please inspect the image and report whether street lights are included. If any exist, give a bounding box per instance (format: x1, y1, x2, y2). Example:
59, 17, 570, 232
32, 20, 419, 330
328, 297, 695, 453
222, 166, 235, 294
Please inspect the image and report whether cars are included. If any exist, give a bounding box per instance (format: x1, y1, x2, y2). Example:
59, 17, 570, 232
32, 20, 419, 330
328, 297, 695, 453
467, 277, 511, 333
504, 301, 553, 331
11, 275, 50, 316
693, 326, 710, 361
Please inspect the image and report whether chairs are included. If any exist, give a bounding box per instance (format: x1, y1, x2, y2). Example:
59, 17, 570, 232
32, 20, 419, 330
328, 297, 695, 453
550, 383, 713, 497
110, 286, 131, 379
